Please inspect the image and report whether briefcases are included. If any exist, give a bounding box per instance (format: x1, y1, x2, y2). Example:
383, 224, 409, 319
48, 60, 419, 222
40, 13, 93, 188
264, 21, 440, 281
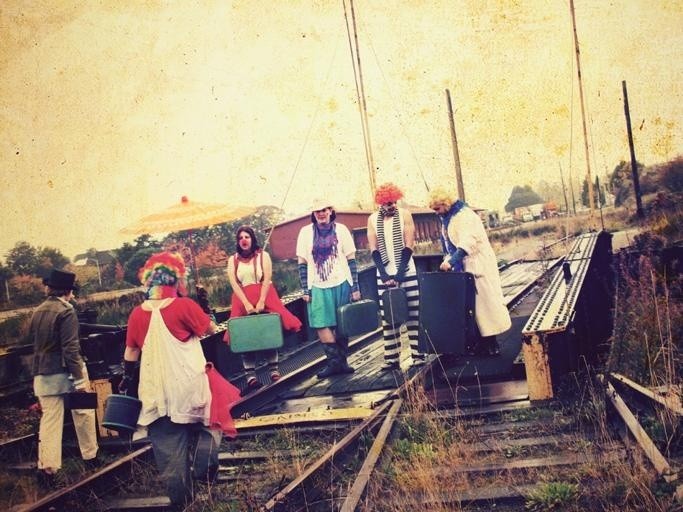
228, 312, 285, 355
336, 298, 378, 338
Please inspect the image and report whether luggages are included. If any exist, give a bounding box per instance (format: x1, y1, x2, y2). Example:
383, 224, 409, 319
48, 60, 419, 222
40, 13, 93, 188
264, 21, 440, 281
418, 270, 476, 354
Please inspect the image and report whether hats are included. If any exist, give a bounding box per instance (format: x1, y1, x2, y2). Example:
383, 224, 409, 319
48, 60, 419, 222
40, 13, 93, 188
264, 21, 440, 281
304, 197, 336, 212
43, 268, 80, 290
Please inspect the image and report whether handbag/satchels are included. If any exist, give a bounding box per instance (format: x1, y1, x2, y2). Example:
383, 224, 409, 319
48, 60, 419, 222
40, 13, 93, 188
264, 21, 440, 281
65, 389, 98, 409
382, 287, 410, 325
100, 393, 142, 431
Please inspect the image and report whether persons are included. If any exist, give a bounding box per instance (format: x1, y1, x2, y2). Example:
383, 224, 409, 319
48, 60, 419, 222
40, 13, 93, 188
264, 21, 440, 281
26, 267, 103, 490
430, 183, 512, 356
293, 195, 363, 378
220, 226, 302, 389
116, 251, 242, 511
365, 182, 429, 371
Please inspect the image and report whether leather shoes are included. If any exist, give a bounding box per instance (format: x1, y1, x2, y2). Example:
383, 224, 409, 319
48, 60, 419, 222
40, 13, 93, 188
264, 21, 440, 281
271, 372, 281, 381
248, 379, 260, 388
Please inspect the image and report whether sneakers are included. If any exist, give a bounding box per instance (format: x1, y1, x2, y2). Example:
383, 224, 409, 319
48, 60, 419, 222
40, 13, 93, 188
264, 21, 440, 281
36, 468, 56, 488
195, 482, 224, 503
84, 458, 110, 474
380, 362, 399, 369
413, 358, 426, 366
465, 344, 499, 357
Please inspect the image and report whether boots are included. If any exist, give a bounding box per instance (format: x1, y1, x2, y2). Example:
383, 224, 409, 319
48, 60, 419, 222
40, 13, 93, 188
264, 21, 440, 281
335, 337, 355, 373
318, 342, 344, 378
